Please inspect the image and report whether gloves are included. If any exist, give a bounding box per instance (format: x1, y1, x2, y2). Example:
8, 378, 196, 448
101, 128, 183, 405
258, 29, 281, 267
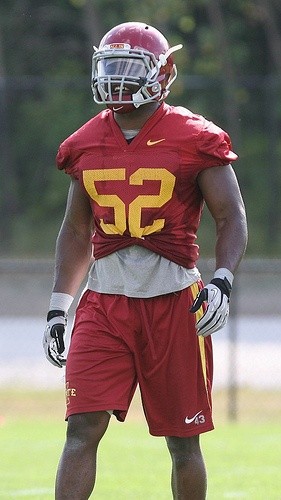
190, 268, 236, 337
41, 292, 76, 368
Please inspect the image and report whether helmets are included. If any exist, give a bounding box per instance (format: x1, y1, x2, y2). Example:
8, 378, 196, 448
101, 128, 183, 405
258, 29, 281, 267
91, 22, 175, 112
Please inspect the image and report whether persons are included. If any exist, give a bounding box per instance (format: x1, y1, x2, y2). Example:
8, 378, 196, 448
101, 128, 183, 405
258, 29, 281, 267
41, 21, 249, 500
